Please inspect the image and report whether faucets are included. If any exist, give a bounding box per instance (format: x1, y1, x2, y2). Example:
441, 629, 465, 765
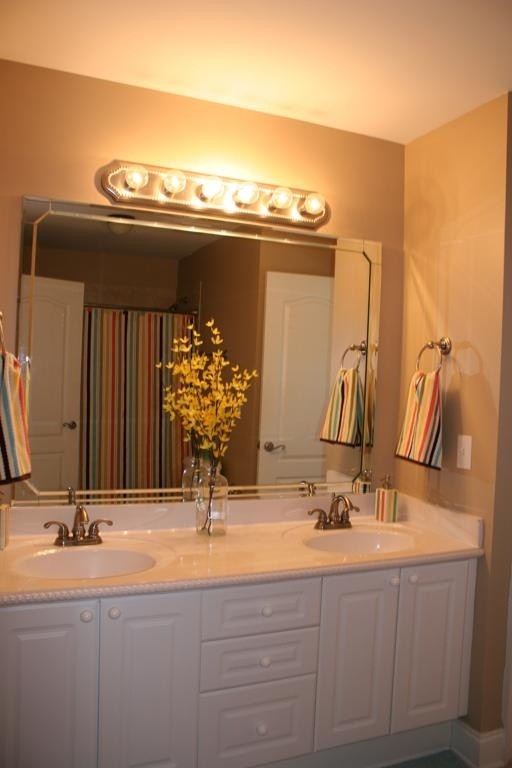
300, 480, 317, 496
66, 483, 77, 504
307, 495, 360, 529
43, 508, 113, 546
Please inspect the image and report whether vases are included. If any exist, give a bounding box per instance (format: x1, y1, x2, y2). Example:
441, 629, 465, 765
180, 455, 198, 504
193, 461, 229, 544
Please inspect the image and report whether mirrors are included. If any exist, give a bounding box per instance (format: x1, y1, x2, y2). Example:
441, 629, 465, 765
10, 194, 384, 510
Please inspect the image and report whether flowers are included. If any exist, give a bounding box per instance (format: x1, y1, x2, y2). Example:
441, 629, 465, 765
153, 316, 260, 535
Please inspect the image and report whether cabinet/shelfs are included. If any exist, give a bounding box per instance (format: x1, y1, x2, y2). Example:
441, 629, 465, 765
312, 560, 477, 767
0, 591, 199, 766
201, 576, 324, 768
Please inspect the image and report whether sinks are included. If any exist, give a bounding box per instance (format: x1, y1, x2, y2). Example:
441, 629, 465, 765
300, 523, 419, 556
9, 544, 161, 583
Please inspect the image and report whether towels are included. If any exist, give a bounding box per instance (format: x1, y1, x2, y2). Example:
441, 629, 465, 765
391, 369, 444, 472
317, 366, 377, 452
1, 352, 33, 485
